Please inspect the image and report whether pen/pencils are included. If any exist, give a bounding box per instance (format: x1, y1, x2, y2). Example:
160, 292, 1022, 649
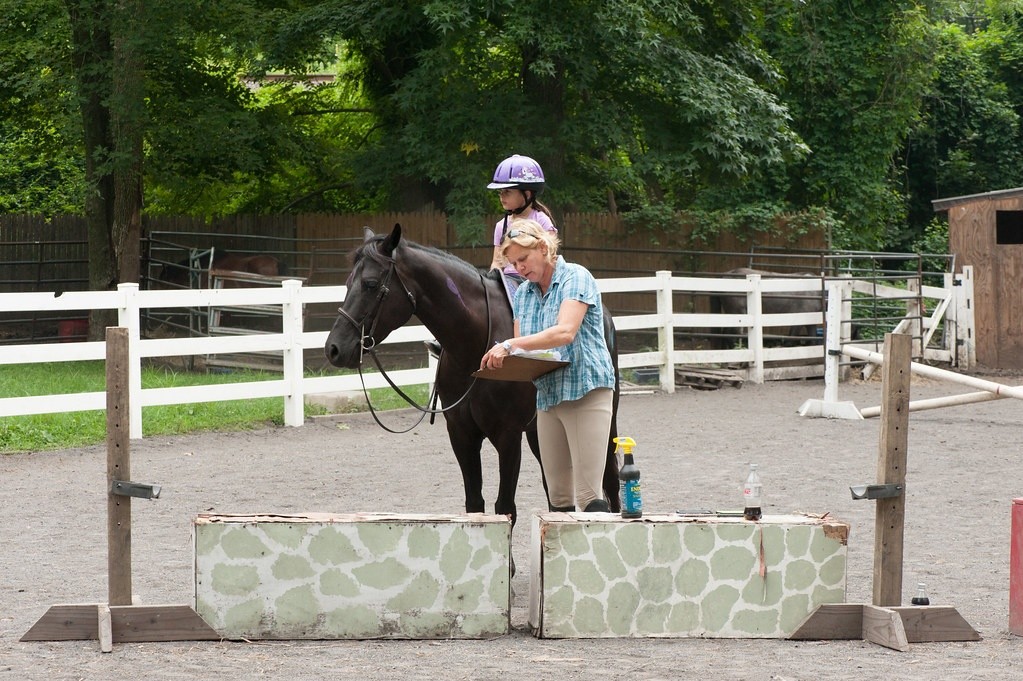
494, 341, 502, 344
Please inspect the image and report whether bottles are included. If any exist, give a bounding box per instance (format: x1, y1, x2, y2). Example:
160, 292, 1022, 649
742, 463, 763, 522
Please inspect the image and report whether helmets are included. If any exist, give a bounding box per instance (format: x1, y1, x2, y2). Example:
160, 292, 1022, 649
486, 155, 545, 189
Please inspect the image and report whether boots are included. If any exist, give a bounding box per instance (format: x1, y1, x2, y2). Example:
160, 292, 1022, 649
547, 507, 575, 512
584, 497, 610, 513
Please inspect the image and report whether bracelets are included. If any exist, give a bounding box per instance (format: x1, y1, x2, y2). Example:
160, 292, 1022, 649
503, 341, 512, 354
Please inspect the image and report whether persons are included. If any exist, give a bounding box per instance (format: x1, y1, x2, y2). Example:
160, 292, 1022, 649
488, 154, 558, 273
481, 220, 616, 512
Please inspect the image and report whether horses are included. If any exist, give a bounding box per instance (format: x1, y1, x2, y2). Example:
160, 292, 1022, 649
325, 223, 620, 514
709, 269, 865, 369
150, 248, 281, 341
